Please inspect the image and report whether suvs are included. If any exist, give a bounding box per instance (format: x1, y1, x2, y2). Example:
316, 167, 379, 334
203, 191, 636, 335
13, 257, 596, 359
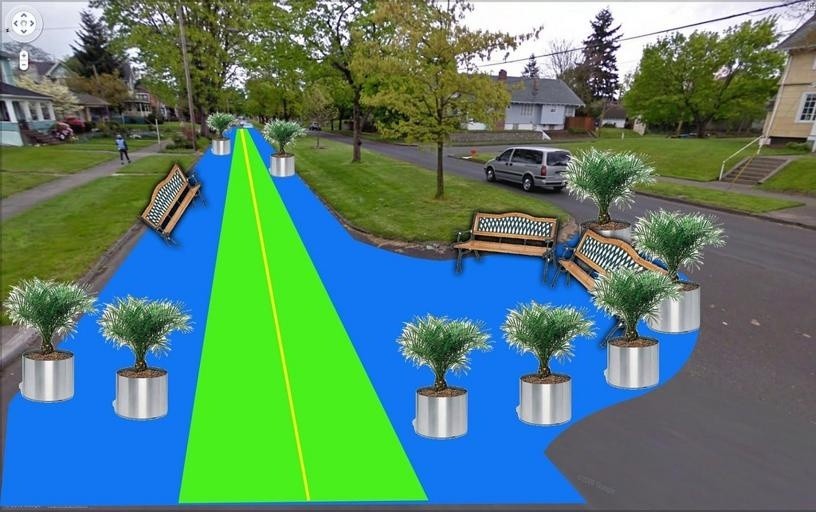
309, 122, 323, 131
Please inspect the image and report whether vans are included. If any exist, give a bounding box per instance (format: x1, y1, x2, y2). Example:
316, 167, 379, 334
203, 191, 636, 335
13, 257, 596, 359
483, 144, 574, 192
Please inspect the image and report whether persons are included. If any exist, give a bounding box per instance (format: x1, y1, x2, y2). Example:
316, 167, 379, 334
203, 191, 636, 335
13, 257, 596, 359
115, 134, 131, 165
